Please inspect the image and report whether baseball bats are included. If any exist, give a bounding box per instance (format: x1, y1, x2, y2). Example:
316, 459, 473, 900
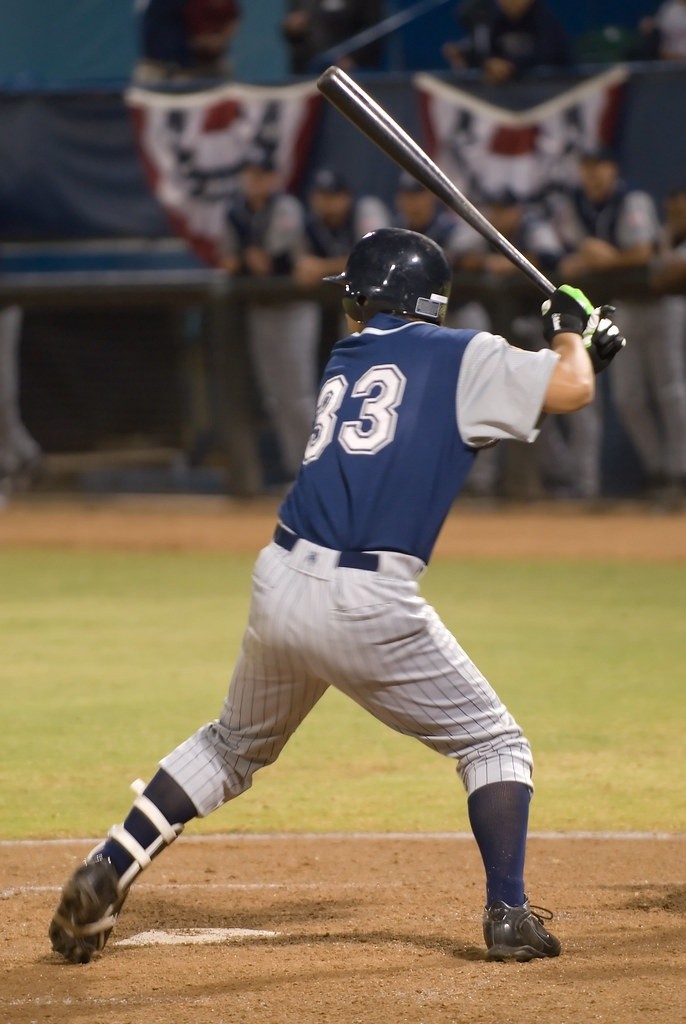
314, 62, 557, 296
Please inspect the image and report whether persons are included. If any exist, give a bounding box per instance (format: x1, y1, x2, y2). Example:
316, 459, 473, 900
136, 0, 685, 89
0, 300, 41, 494
49, 224, 628, 967
230, 152, 686, 501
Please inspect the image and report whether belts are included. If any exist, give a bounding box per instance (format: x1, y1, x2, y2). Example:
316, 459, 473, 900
273, 524, 379, 571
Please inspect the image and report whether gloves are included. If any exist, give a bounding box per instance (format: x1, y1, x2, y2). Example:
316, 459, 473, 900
542, 284, 594, 343
581, 303, 628, 374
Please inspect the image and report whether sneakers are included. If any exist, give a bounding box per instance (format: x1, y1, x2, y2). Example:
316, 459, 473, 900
483, 892, 561, 960
48, 861, 126, 964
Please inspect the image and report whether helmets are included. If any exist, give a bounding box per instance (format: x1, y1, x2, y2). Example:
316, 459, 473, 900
323, 226, 450, 325
